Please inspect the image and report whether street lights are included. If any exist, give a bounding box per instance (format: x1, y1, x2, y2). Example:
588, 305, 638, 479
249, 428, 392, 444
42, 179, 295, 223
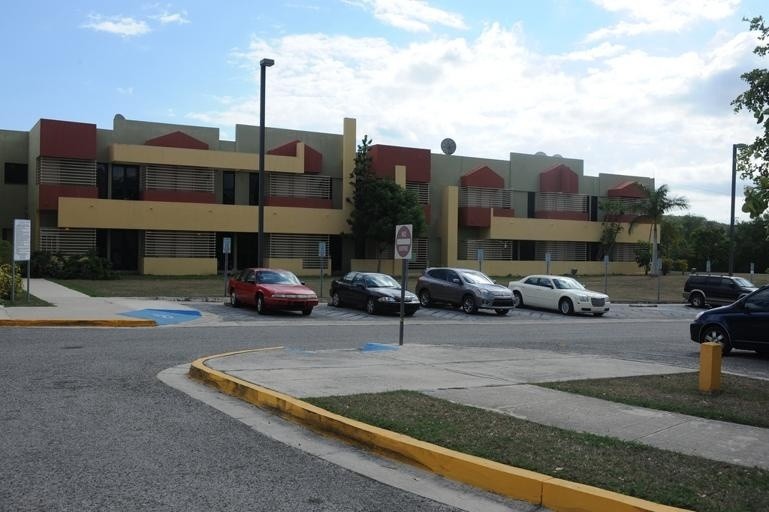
727, 142, 751, 274
256, 57, 277, 267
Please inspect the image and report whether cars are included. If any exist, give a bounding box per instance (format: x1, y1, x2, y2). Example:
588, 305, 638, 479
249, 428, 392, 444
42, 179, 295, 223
224, 264, 320, 318
328, 271, 422, 319
689, 284, 769, 356
503, 273, 611, 319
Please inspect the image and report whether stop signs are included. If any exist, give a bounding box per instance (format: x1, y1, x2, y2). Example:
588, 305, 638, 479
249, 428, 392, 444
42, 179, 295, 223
393, 223, 413, 260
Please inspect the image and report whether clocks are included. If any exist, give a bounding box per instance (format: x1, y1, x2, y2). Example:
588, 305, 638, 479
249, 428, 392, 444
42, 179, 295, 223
441, 138, 456, 154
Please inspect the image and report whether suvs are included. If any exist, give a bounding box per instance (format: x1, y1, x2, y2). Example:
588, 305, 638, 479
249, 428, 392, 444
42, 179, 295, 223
680, 271, 761, 307
414, 264, 517, 317
